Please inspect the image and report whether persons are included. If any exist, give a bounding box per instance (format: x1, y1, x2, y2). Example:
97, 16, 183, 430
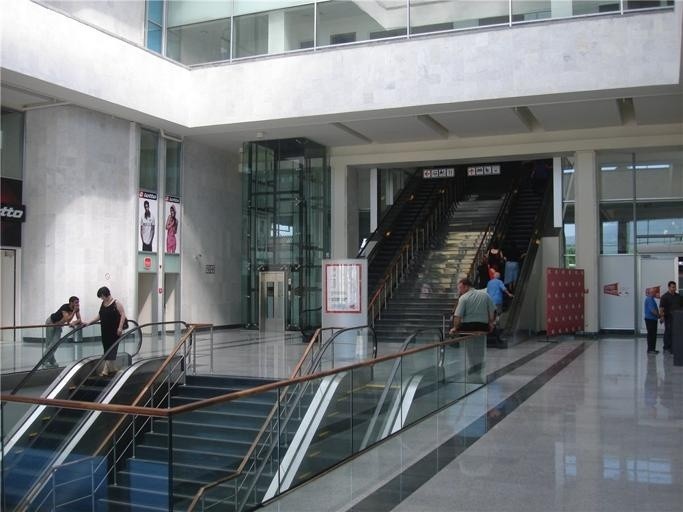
141, 201, 156, 251
166, 205, 178, 254
659, 281, 680, 350
449, 279, 498, 374
645, 288, 660, 354
43, 296, 82, 369
645, 354, 657, 417
81, 286, 126, 377
484, 235, 527, 334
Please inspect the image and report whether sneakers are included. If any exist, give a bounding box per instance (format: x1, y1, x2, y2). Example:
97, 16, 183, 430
647, 350, 660, 354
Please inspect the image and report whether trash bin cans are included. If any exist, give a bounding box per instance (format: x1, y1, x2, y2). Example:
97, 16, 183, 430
73, 324, 82, 342
356, 329, 374, 357
